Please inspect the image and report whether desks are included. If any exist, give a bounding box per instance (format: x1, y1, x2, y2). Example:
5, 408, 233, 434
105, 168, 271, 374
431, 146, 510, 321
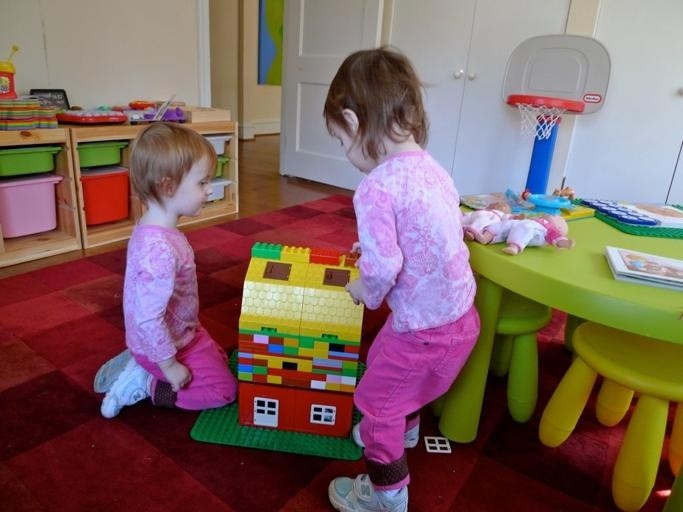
438, 202, 682, 444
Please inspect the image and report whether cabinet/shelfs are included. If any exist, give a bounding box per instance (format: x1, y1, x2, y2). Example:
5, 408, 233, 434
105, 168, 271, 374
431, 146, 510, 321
0, 106, 239, 265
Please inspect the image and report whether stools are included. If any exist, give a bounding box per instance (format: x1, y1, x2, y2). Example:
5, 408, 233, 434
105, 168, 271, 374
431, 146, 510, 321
536, 321, 683, 512
496, 288, 552, 422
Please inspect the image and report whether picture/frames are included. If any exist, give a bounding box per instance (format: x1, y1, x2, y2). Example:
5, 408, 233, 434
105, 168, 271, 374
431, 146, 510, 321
30, 88, 70, 109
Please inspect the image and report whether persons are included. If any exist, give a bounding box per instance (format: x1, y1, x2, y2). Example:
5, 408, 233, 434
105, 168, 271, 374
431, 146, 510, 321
323, 48, 481, 512
92, 123, 236, 418
462, 203, 575, 256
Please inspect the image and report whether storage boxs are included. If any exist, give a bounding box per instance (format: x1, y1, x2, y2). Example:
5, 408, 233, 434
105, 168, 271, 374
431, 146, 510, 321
0, 134, 232, 239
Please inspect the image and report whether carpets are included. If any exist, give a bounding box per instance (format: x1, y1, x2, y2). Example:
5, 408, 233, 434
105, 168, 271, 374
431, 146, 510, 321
0, 196, 683, 512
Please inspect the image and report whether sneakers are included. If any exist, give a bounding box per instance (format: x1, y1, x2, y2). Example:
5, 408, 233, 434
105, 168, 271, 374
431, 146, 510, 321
352, 414, 420, 448
328, 473, 409, 512
94, 349, 154, 419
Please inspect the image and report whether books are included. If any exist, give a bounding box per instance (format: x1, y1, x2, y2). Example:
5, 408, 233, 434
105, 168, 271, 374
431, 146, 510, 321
604, 245, 683, 291
